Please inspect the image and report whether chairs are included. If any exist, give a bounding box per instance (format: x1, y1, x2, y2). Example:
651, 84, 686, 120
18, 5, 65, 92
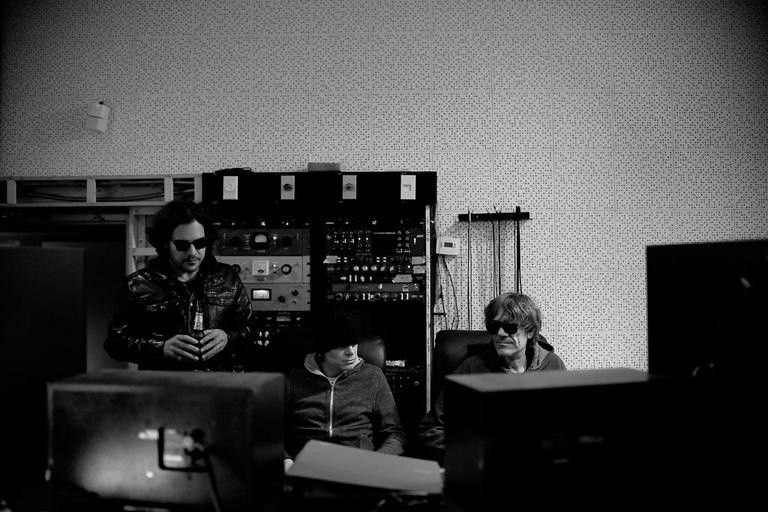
431, 329, 546, 409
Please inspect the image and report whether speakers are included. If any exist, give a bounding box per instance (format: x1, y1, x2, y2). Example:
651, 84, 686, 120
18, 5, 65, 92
646, 238, 768, 511
42, 369, 287, 512
442, 367, 649, 512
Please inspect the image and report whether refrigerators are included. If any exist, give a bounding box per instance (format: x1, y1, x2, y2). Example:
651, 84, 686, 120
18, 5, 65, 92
206, 169, 434, 419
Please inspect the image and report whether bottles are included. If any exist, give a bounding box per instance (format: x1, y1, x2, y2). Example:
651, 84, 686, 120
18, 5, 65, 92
359, 335, 385, 368
327, 223, 414, 303
189, 312, 207, 372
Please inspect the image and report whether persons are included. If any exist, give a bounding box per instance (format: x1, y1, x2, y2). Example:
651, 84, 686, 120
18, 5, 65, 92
418, 292, 566, 461
104, 200, 256, 370
284, 312, 409, 473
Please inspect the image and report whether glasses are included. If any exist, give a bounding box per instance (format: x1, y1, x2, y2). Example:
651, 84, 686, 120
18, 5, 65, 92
169, 237, 207, 251
486, 318, 524, 335
